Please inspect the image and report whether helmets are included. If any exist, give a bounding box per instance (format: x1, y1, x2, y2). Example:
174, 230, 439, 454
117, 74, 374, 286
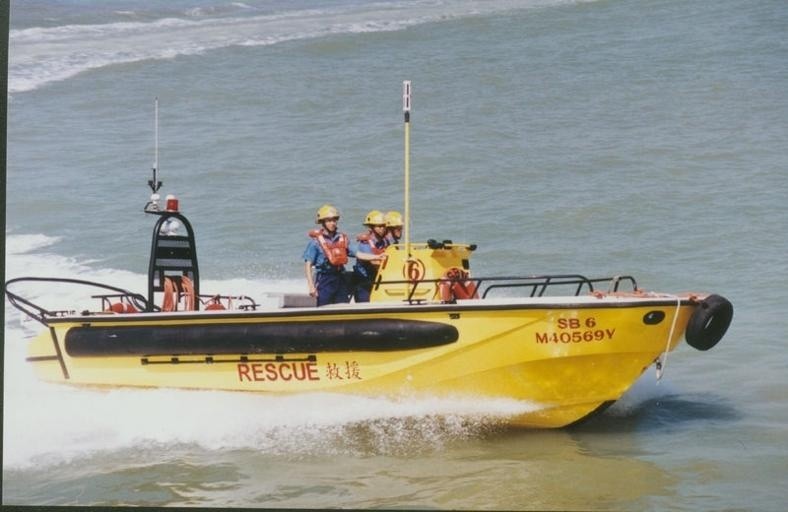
364, 211, 387, 226
386, 211, 404, 228
314, 204, 340, 223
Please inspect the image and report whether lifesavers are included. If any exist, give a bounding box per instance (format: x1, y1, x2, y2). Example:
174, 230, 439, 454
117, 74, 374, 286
105, 303, 138, 313
439, 267, 479, 301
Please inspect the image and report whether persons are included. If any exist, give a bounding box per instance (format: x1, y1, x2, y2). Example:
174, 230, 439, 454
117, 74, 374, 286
353, 209, 390, 303
304, 206, 387, 308
385, 211, 403, 252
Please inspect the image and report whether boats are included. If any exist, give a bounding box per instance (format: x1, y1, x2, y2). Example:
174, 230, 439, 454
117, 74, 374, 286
4, 80, 712, 429
